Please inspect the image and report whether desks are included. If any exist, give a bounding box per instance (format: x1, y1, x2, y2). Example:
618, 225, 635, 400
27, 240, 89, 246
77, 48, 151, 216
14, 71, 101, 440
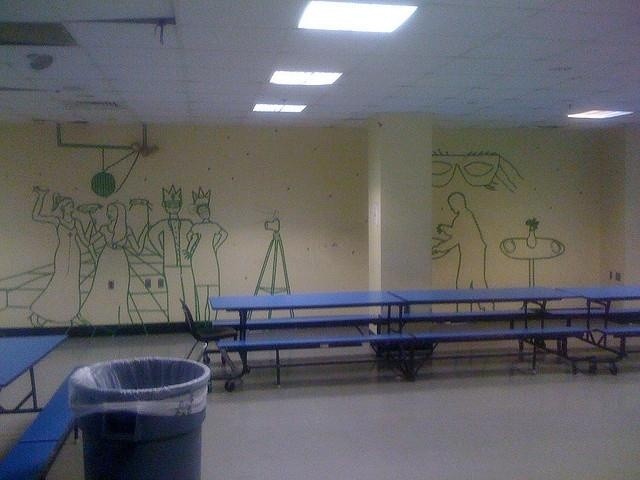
0, 334, 70, 414
569, 283, 640, 356
208, 290, 410, 380
388, 286, 582, 381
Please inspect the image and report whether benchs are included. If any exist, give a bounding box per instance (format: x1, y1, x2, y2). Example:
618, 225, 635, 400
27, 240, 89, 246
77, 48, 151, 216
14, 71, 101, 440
216, 332, 414, 385
378, 307, 543, 335
211, 312, 382, 341
592, 325, 640, 361
410, 325, 591, 382
0, 366, 81, 480
544, 306, 640, 327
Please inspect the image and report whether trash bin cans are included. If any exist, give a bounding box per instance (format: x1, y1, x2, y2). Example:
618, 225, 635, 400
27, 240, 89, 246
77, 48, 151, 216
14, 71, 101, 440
70, 356, 211, 480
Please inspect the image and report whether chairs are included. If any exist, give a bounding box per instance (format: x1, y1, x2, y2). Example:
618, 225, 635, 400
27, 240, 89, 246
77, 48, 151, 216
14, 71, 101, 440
179, 297, 239, 364
